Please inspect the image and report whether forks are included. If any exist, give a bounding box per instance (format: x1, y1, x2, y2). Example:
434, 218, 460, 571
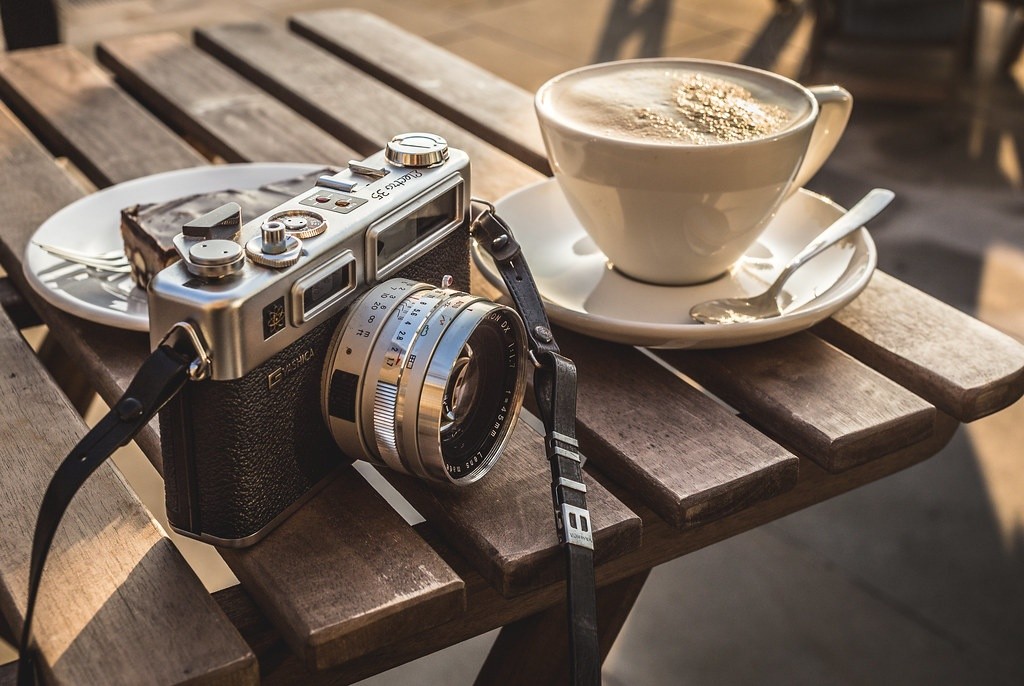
30, 240, 132, 273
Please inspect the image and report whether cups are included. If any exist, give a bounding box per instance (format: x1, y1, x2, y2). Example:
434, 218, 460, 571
532, 56, 853, 287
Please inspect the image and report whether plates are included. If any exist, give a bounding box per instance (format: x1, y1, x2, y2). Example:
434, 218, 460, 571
470, 177, 877, 349
23, 161, 350, 332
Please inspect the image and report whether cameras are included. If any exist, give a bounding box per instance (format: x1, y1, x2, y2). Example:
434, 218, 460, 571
147, 133, 529, 548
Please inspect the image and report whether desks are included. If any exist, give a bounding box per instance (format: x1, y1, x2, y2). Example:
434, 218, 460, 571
1, 5, 1024, 686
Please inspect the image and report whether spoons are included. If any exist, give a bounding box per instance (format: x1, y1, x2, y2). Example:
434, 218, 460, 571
687, 188, 896, 324
